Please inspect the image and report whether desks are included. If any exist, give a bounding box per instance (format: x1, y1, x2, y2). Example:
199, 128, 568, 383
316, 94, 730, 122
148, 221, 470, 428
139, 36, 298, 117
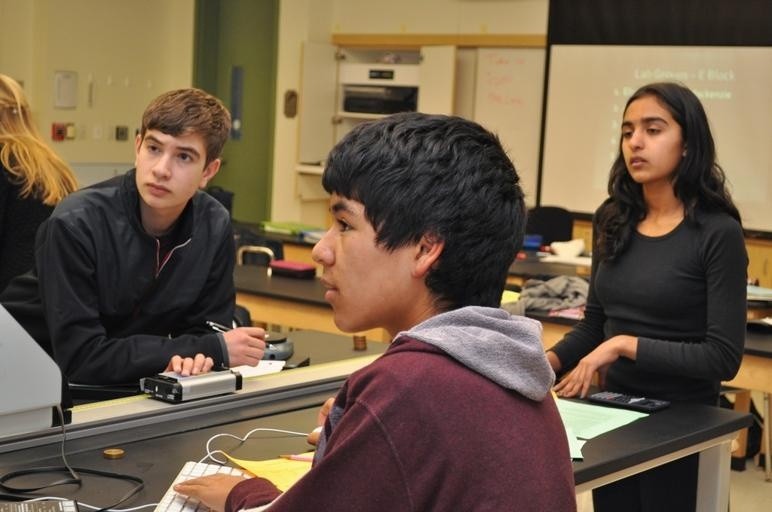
1, 221, 771, 511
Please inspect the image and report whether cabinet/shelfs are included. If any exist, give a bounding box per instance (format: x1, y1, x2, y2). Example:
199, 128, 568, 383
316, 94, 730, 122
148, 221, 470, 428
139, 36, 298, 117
298, 41, 457, 166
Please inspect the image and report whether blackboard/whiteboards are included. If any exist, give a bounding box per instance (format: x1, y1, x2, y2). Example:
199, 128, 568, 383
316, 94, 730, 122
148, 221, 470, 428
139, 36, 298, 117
471, 47, 547, 211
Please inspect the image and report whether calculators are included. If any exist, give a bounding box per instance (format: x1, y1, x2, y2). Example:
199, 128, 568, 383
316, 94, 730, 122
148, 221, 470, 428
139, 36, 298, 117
586, 390, 671, 412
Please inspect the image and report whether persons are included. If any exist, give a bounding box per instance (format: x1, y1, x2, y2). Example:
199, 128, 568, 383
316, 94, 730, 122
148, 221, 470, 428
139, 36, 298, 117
545, 81, 750, 409
170, 110, 580, 512
0, 71, 83, 290
38, 87, 267, 383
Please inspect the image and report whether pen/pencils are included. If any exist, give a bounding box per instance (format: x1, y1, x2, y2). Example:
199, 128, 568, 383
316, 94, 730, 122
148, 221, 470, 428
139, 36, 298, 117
278, 455, 313, 462
206, 321, 278, 351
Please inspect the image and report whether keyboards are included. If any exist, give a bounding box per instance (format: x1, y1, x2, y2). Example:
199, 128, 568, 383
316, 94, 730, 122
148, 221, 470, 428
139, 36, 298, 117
151, 459, 257, 512
